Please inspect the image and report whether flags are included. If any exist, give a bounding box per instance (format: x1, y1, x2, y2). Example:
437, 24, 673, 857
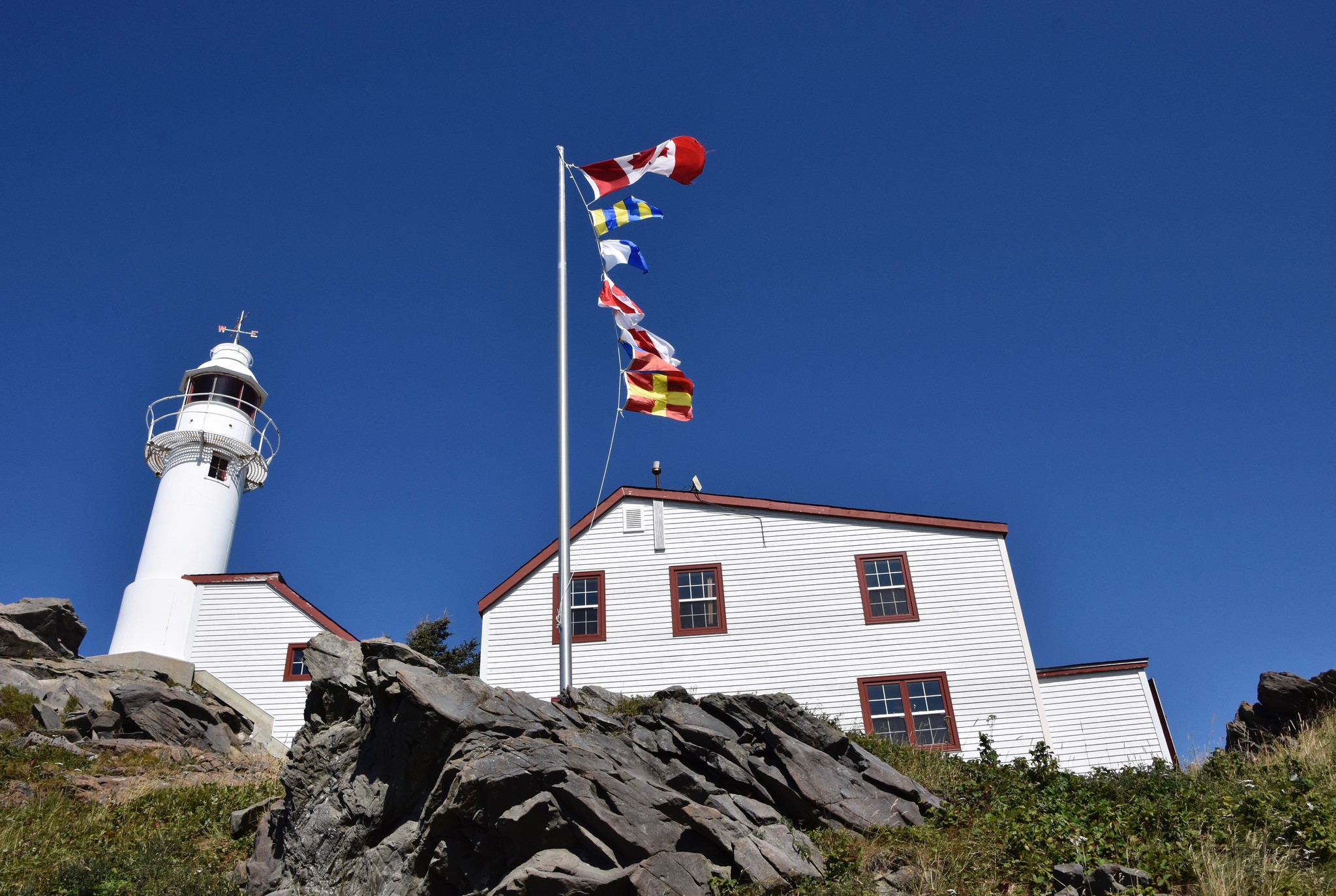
574, 135, 709, 422
